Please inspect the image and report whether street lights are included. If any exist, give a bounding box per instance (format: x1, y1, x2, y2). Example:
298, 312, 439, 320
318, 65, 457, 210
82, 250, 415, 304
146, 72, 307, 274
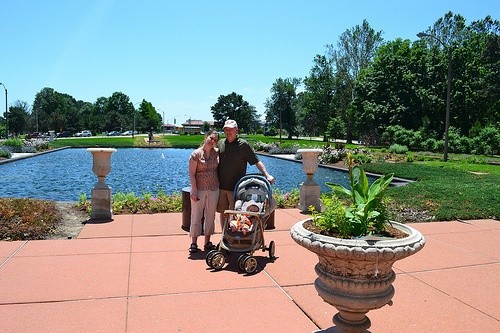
157, 108, 164, 136
416, 31, 452, 162
133, 102, 145, 139
0, 83, 8, 139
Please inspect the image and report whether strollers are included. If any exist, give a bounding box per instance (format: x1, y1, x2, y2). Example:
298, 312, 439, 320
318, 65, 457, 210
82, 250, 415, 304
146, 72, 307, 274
204, 172, 276, 275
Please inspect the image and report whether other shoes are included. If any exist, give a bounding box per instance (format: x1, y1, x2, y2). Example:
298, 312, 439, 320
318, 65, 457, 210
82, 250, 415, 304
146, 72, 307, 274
189, 243, 198, 253
204, 242, 216, 251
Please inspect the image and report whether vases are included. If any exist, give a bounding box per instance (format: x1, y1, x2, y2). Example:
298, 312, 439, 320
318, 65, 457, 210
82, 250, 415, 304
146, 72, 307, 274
297, 148, 324, 175
86, 148, 117, 178
289, 218, 425, 313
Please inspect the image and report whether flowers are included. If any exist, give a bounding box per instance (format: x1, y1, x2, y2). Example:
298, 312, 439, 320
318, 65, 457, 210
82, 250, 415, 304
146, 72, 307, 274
307, 147, 400, 238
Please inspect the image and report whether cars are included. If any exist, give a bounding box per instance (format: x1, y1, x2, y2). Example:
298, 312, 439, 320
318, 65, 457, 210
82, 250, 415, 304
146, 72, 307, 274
56, 131, 72, 137
28, 132, 44, 138
75, 130, 91, 137
121, 130, 132, 136
108, 131, 120, 136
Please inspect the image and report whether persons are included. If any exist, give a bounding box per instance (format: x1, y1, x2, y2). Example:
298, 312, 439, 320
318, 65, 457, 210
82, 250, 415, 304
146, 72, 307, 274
230, 204, 259, 235
194, 119, 274, 232
188, 131, 220, 252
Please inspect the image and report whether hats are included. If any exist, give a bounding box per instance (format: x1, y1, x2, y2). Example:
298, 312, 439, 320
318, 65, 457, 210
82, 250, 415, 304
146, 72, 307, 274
223, 120, 237, 129
